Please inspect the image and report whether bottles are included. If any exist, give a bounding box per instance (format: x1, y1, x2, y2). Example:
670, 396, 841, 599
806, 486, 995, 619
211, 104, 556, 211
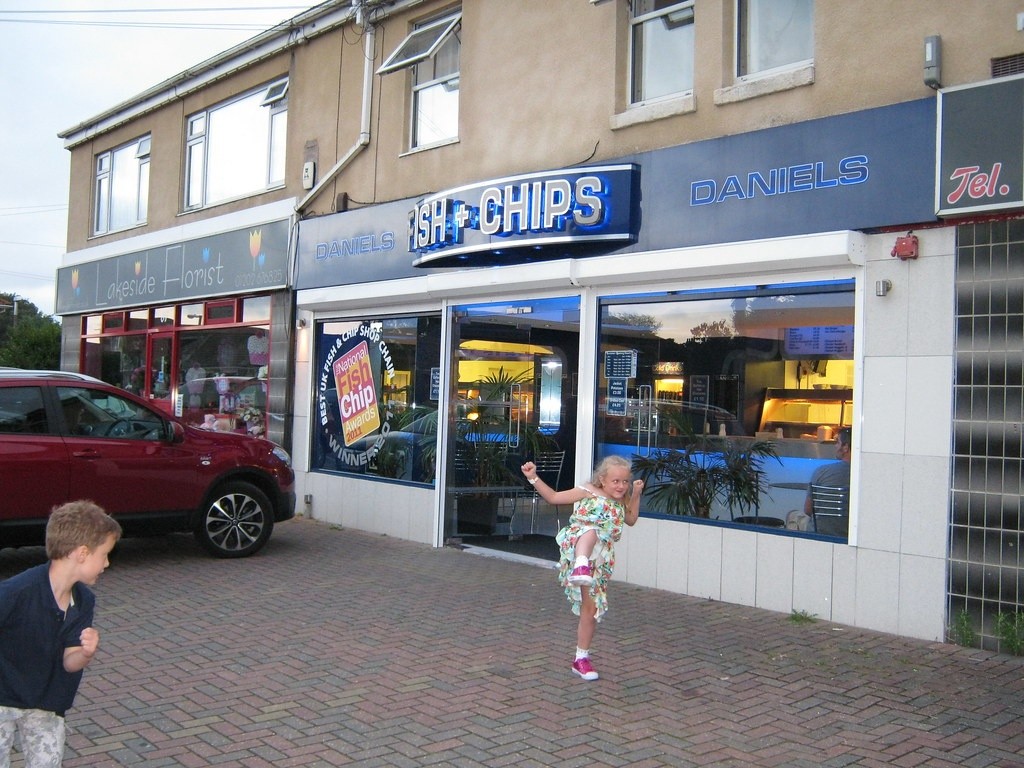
659, 390, 682, 401
777, 428, 783, 438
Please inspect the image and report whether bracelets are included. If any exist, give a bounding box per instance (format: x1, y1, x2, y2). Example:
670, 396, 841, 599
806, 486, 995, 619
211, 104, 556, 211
529, 476, 539, 484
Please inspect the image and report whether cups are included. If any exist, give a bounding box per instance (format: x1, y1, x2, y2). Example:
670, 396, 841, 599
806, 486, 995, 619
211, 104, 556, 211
719, 424, 726, 436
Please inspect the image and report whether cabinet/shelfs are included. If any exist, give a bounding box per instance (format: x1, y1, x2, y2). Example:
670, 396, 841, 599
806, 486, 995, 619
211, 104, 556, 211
458, 346, 534, 424
757, 385, 853, 437
383, 370, 414, 418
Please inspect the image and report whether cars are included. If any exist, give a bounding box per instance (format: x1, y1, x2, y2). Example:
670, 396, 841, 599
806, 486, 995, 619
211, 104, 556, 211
333, 402, 527, 487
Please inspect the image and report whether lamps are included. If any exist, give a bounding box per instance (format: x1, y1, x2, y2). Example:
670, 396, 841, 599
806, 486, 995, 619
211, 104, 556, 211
914, 32, 952, 87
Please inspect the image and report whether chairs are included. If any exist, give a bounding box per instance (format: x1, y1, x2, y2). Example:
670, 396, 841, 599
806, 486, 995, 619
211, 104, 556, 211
507, 449, 567, 539
805, 482, 851, 538
473, 433, 513, 526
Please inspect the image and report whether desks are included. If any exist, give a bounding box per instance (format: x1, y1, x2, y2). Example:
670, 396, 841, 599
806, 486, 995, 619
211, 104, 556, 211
724, 514, 787, 529
441, 485, 525, 537
768, 479, 812, 492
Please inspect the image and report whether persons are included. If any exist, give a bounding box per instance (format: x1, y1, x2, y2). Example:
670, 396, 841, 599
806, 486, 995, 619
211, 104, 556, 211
0, 501, 123, 768
521, 454, 644, 680
803, 426, 851, 537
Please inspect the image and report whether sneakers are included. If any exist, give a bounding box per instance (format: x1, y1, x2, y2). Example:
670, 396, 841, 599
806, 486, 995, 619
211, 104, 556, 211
572, 657, 599, 680
568, 566, 593, 586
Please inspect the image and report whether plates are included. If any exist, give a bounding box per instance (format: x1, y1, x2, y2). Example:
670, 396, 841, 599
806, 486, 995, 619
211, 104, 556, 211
813, 384, 851, 390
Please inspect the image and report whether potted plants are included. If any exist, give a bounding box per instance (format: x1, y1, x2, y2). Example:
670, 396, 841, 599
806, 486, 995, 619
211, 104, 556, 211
376, 363, 559, 536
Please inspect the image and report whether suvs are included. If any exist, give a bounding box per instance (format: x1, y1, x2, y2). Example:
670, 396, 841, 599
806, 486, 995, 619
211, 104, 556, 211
0, 367, 296, 558
598, 398, 748, 458
149, 377, 268, 427
